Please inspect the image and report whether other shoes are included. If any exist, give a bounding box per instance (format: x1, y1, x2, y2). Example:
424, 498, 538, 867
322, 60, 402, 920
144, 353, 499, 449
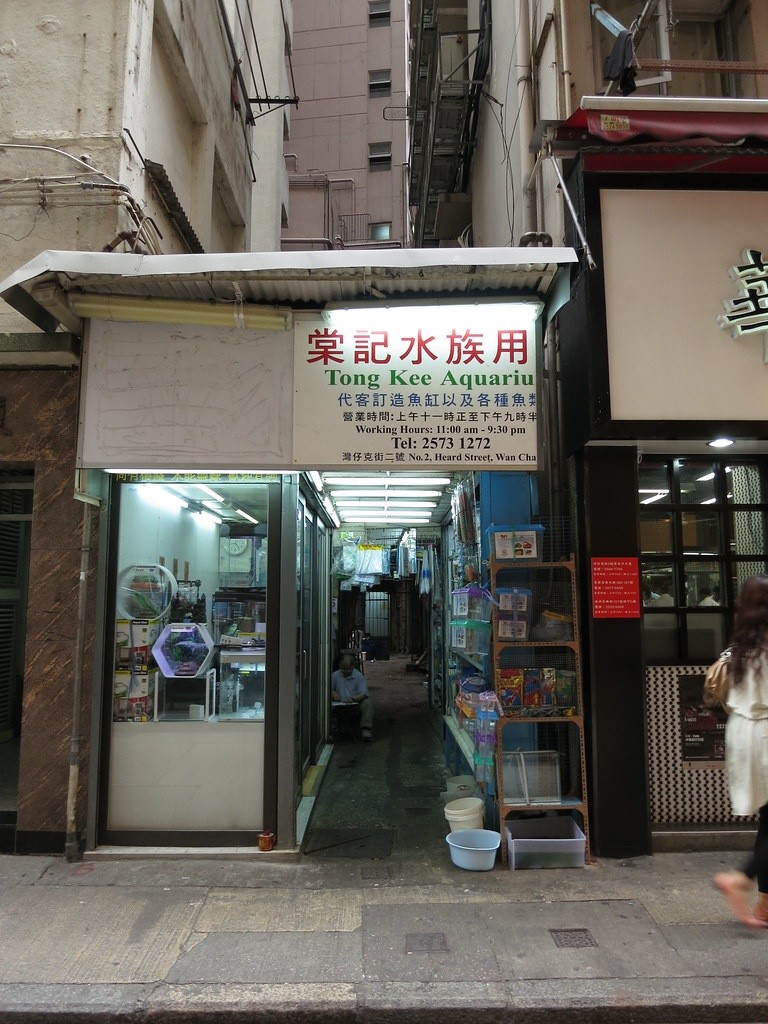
361, 730, 372, 738
713, 869, 768, 927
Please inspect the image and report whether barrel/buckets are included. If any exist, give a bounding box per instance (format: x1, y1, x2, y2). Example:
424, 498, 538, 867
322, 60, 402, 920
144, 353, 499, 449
444, 798, 487, 832
446, 775, 476, 797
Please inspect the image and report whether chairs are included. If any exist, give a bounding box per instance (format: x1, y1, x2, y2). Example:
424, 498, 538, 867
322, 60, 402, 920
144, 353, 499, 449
341, 630, 365, 675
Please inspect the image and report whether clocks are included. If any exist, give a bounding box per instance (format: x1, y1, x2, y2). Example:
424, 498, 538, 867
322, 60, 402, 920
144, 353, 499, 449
222, 538, 248, 556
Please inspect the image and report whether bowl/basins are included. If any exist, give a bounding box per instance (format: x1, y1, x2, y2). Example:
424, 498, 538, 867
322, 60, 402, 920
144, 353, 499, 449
445, 828, 501, 870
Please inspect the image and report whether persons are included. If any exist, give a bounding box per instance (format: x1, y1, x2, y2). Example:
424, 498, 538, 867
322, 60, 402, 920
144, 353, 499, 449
711, 575, 768, 930
330, 654, 367, 744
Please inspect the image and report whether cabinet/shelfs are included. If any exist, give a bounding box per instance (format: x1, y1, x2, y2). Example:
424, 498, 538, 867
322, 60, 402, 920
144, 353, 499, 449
428, 471, 540, 830
489, 554, 591, 865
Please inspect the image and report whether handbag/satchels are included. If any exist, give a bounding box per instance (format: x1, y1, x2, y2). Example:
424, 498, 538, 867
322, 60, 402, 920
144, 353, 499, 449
703, 646, 733, 714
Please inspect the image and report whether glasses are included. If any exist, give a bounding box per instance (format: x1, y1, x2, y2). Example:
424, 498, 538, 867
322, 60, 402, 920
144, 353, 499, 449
340, 666, 352, 672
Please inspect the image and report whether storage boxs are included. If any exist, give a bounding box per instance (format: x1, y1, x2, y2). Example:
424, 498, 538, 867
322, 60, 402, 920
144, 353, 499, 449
498, 668, 556, 709
114, 618, 163, 721
449, 585, 534, 654
504, 816, 587, 871
485, 525, 545, 563
473, 690, 499, 783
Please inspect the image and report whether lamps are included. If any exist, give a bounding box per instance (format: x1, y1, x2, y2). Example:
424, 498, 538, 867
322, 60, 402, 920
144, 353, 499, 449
196, 484, 263, 525
696, 490, 733, 504
638, 476, 695, 495
694, 467, 732, 482
